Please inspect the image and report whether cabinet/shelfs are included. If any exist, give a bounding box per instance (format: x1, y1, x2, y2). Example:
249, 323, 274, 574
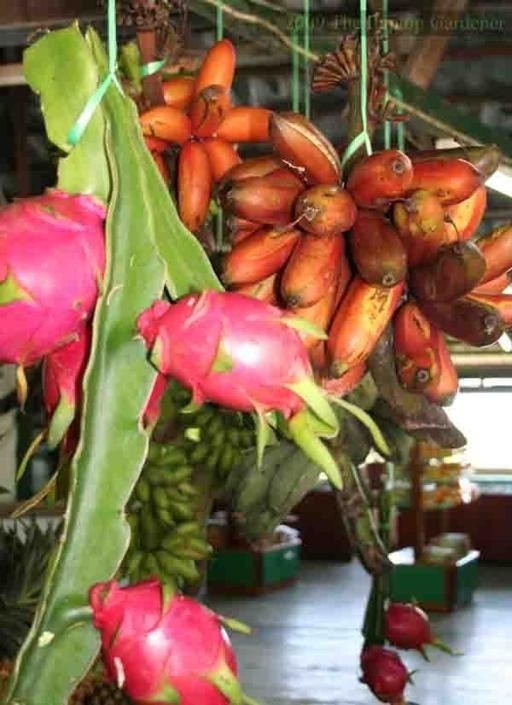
196, 485, 304, 602
390, 443, 482, 616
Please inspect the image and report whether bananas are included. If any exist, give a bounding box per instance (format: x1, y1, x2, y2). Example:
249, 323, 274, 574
118, 39, 512, 586
